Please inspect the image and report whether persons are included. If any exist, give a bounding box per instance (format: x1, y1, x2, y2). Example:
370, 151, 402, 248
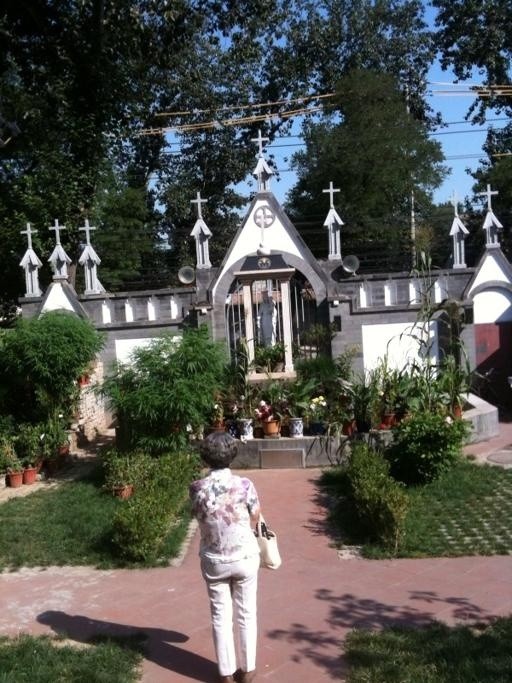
189, 432, 261, 683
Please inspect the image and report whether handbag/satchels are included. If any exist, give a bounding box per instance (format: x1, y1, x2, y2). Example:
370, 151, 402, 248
254, 518, 282, 570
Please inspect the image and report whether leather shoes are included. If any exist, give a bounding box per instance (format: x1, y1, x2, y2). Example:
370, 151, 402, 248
221, 670, 255, 683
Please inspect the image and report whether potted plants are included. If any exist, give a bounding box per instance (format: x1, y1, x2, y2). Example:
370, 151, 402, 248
84, 307, 494, 502
1, 307, 109, 488
